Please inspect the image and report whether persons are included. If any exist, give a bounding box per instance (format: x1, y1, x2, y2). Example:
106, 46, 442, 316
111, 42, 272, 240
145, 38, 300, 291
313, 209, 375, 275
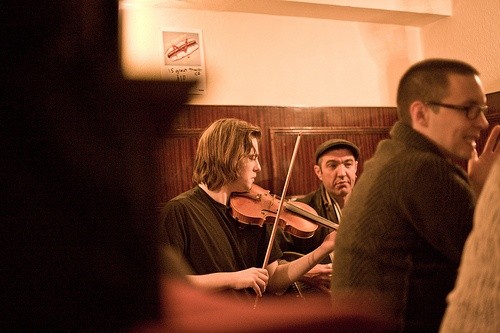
330, 59, 500, 333
278, 140, 360, 298
158, 118, 338, 300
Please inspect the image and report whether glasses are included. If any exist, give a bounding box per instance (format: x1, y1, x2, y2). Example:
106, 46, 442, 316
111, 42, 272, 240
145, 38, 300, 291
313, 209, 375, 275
425, 102, 488, 120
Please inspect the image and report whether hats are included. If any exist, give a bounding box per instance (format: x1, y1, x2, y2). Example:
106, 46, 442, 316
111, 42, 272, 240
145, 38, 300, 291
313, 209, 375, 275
316, 139, 359, 165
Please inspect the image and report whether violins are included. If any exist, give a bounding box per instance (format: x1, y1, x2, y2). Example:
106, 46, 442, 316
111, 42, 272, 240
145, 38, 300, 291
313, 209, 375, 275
230, 183, 340, 239
